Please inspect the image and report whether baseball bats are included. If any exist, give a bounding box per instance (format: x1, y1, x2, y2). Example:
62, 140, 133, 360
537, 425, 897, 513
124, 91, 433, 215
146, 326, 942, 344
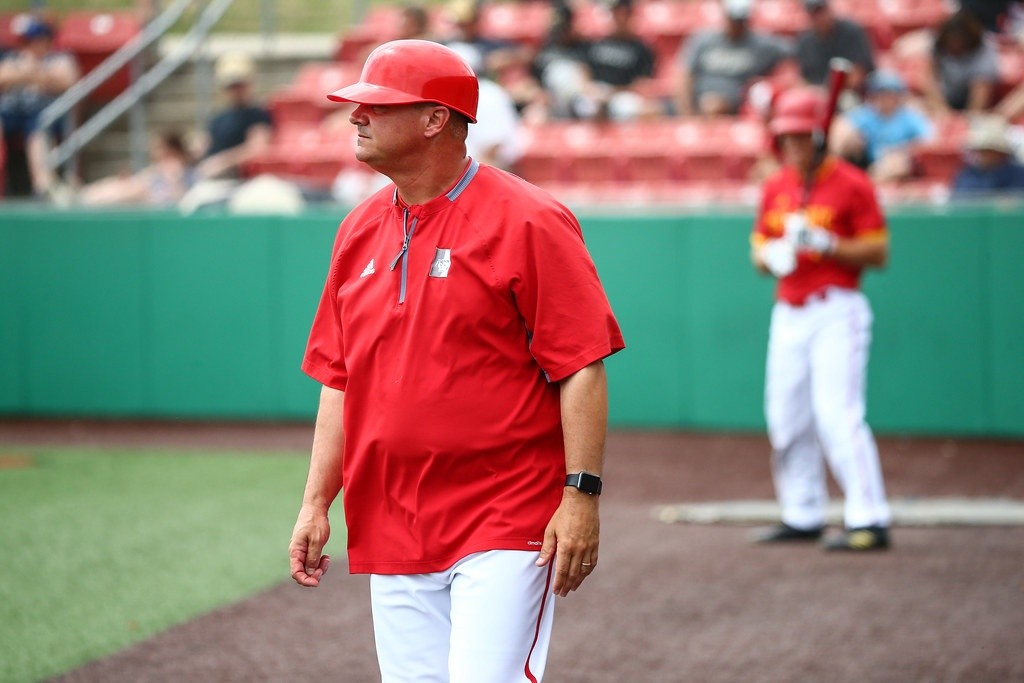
801, 56, 851, 207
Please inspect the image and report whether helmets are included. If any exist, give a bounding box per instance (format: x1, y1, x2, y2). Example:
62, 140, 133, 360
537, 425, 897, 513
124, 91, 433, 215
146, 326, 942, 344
326, 41, 477, 125
768, 86, 831, 163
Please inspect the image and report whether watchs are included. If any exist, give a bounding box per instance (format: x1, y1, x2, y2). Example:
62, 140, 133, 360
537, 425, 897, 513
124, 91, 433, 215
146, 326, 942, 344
565, 471, 602, 496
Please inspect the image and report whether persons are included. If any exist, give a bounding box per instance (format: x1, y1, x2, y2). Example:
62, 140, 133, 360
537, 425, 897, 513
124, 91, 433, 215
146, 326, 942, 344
752, 90, 892, 551
0, 16, 74, 174
141, 56, 268, 209
289, 39, 627, 683
675, 0, 1024, 199
396, 0, 677, 120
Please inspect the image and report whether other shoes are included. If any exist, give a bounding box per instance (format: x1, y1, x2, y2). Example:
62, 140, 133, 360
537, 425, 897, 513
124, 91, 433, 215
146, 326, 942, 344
752, 523, 824, 545
825, 526, 890, 552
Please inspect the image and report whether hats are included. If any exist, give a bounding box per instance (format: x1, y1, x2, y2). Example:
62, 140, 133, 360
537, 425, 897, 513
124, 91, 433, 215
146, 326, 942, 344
214, 53, 255, 86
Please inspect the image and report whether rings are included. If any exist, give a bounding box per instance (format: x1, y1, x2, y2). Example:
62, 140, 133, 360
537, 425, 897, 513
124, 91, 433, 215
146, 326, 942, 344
582, 563, 591, 566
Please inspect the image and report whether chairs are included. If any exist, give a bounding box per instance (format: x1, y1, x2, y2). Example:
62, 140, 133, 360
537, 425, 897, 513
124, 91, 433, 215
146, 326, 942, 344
1, 0, 1023, 211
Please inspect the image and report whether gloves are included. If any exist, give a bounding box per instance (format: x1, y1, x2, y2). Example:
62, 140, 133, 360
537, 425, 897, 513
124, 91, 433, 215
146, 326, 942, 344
785, 215, 833, 255
766, 240, 797, 279
